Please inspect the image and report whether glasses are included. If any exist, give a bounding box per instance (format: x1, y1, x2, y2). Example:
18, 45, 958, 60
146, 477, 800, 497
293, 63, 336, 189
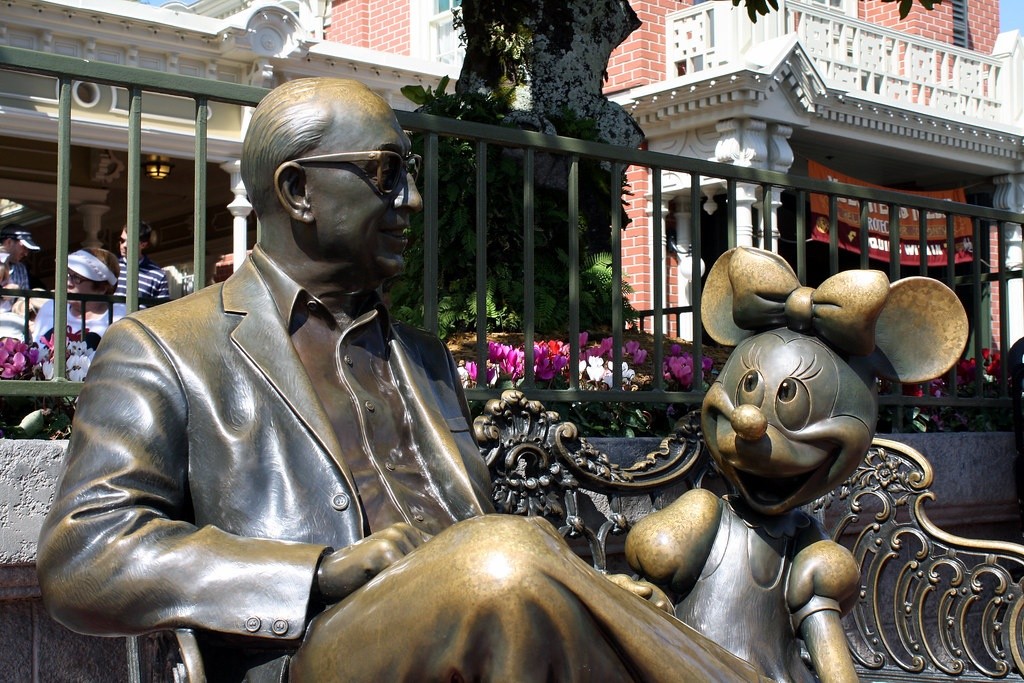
119, 236, 127, 243
67, 273, 95, 285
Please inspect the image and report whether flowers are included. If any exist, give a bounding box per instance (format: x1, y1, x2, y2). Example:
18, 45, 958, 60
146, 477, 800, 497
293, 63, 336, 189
454, 329, 718, 438
871, 345, 1019, 433
0, 326, 95, 434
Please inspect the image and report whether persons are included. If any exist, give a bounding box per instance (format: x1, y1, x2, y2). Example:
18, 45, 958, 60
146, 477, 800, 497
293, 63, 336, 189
35, 78, 784, 683
0, 218, 171, 411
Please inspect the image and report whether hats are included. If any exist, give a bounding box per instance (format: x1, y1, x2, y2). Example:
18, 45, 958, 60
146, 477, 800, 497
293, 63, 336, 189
0, 253, 11, 264
0, 230, 40, 252
67, 249, 118, 286
